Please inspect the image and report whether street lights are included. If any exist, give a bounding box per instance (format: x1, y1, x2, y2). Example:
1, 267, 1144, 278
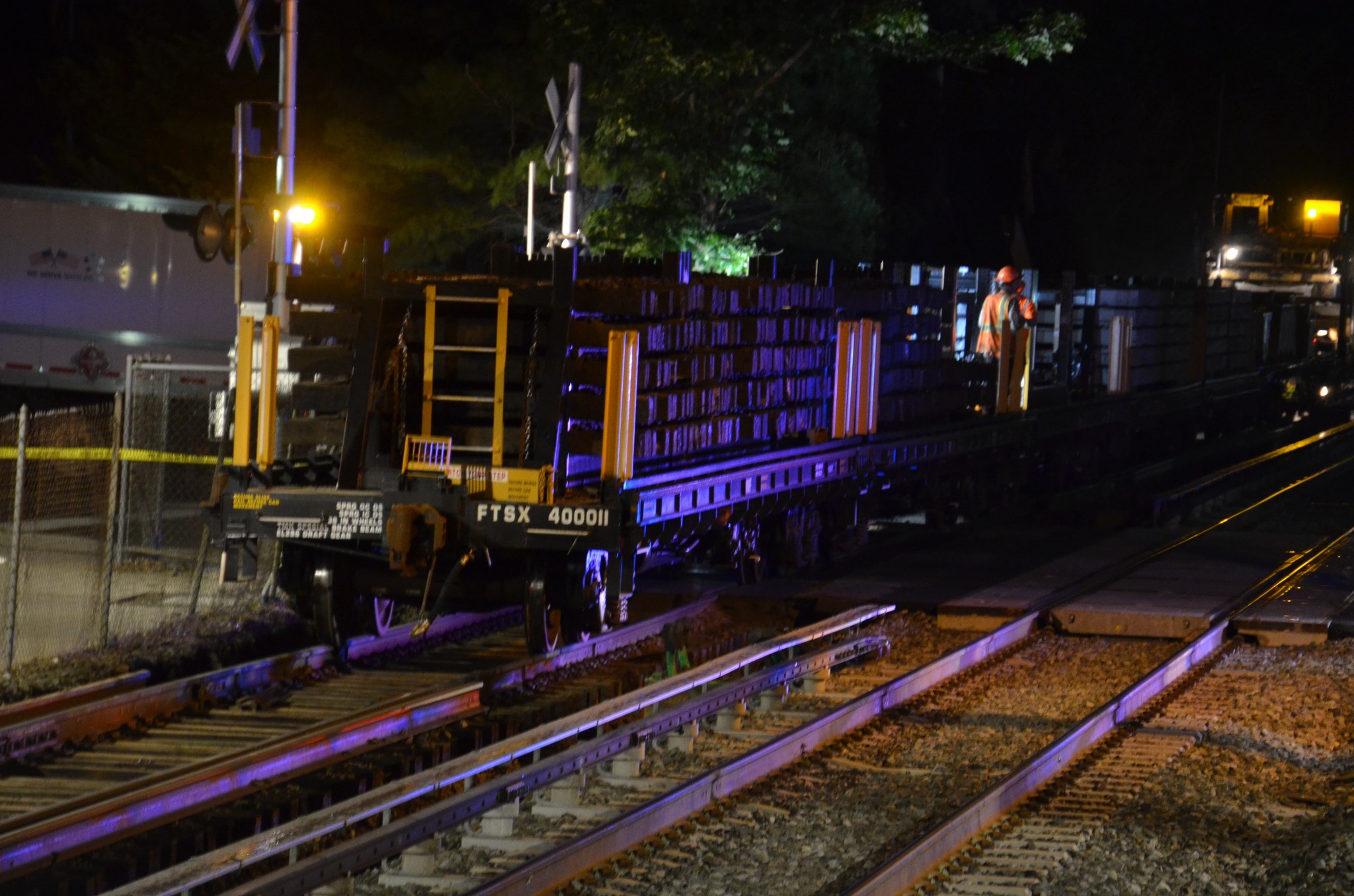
1304, 207, 1316, 239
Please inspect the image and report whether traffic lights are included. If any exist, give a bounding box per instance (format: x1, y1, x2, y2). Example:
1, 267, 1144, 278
194, 209, 225, 265
218, 212, 257, 262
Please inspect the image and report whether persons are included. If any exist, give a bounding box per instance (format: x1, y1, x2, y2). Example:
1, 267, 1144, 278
975, 265, 1038, 416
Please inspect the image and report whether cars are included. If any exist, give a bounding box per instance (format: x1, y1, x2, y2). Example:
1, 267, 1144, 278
1277, 360, 1349, 420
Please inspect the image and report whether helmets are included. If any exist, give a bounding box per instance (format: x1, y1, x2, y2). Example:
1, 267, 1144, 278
995, 266, 1019, 283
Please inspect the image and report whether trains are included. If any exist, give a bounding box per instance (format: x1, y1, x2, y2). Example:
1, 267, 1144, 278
240, 261, 1328, 654
